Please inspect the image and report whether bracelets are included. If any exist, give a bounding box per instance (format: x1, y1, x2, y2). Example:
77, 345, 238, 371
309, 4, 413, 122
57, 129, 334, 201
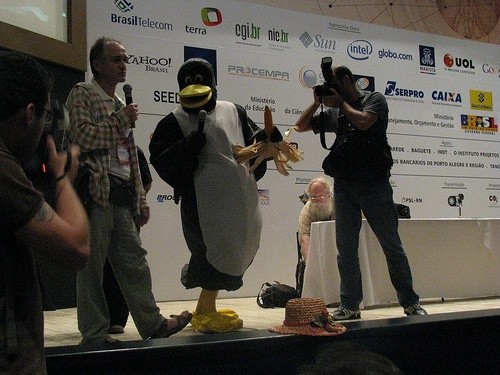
312, 104, 317, 110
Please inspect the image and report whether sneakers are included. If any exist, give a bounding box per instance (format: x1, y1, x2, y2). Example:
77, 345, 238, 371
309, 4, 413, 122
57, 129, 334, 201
403, 303, 428, 318
330, 302, 361, 320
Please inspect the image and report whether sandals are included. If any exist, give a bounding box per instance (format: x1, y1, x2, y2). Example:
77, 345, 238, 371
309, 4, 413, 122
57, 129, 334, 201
151, 311, 193, 340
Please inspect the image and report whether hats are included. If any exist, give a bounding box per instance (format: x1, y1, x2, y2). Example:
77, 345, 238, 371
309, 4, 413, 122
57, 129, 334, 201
269, 298, 345, 335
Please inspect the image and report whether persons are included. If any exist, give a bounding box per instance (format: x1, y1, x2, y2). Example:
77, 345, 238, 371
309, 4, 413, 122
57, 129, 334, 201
296, 178, 336, 309
1, 50, 91, 375
58, 36, 194, 347
294, 65, 427, 321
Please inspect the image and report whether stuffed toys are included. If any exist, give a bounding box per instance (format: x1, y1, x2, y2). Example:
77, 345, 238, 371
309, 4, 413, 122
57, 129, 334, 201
149, 57, 283, 333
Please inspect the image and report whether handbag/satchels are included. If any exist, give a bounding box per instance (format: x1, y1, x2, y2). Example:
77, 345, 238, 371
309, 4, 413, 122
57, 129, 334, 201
256, 281, 298, 309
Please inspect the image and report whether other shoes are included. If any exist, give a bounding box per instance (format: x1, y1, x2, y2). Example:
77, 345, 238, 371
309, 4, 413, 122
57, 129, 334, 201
109, 326, 124, 334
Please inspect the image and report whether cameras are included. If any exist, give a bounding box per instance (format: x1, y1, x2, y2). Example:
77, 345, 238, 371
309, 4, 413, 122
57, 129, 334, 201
22, 127, 70, 178
313, 57, 338, 97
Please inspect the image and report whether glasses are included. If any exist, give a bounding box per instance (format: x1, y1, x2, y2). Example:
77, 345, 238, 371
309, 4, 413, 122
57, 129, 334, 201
310, 193, 327, 202
23, 103, 54, 124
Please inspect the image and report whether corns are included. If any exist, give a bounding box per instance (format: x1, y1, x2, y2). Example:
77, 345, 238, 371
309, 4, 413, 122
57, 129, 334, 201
264, 105, 273, 140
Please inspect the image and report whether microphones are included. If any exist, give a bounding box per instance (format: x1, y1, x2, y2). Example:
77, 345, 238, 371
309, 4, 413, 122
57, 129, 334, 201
198, 110, 207, 132
123, 84, 136, 128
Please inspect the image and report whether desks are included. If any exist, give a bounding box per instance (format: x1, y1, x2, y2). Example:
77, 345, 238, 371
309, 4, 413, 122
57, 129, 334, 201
300, 217, 500, 311
